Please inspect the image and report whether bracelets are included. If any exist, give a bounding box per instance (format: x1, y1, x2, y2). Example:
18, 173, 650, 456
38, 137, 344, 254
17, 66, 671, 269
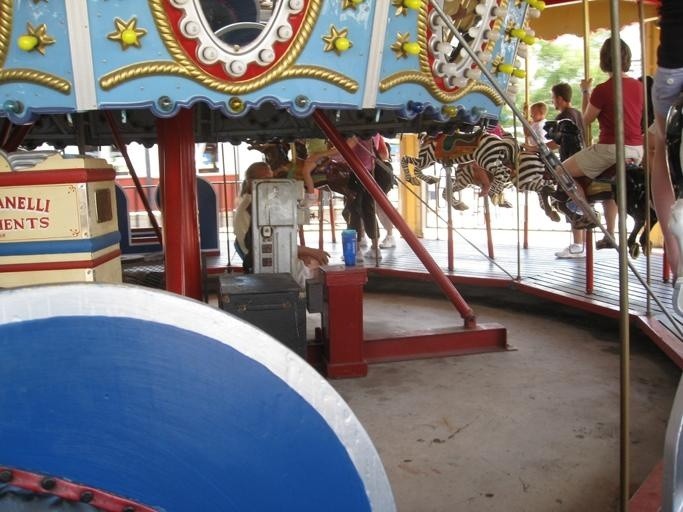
580, 88, 591, 96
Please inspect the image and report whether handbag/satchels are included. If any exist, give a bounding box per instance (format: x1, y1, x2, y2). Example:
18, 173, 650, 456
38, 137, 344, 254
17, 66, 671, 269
347, 136, 375, 193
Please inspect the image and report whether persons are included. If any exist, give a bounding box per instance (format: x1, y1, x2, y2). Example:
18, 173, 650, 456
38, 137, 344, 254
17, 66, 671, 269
522, 102, 548, 156
309, 133, 388, 262
653, 8, 682, 313
296, 137, 329, 210
231, 162, 330, 299
554, 37, 643, 250
357, 142, 396, 249
637, 75, 653, 133
521, 82, 592, 258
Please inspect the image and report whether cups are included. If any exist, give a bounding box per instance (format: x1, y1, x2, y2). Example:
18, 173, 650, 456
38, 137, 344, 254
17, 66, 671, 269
341, 230, 358, 266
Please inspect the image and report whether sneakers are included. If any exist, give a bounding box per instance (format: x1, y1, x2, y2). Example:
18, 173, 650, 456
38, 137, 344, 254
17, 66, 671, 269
379, 236, 395, 247
554, 244, 586, 258
596, 235, 617, 248
363, 245, 383, 261
340, 250, 364, 262
571, 210, 601, 231
360, 237, 368, 246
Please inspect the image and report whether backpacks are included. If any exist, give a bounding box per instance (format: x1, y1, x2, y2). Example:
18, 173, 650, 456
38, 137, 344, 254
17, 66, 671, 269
371, 136, 398, 196
